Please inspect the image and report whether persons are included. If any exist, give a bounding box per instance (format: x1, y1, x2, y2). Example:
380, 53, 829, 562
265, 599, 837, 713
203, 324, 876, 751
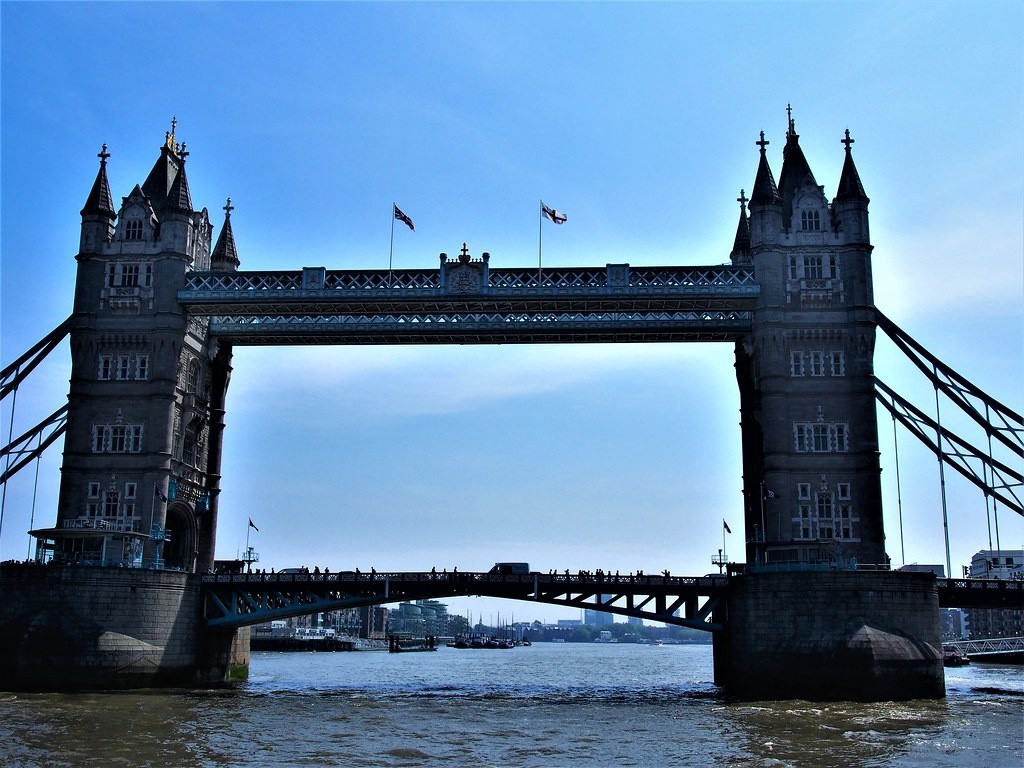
444, 569, 446, 577
249, 568, 274, 580
432, 567, 436, 580
596, 569, 619, 582
565, 569, 569, 574
299, 566, 329, 580
356, 568, 360, 580
549, 569, 557, 580
208, 566, 227, 574
371, 567, 376, 580
662, 569, 670, 580
630, 570, 643, 582
578, 570, 593, 580
1008, 571, 1024, 580
454, 567, 457, 572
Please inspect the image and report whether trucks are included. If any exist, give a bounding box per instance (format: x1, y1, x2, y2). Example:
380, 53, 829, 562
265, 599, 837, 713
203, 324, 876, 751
488, 562, 530, 575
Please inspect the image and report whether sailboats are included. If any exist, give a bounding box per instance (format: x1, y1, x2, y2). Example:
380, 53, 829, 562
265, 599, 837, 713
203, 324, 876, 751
447, 609, 533, 648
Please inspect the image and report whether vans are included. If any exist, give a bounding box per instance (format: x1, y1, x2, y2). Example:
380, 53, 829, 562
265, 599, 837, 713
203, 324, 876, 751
277, 568, 309, 580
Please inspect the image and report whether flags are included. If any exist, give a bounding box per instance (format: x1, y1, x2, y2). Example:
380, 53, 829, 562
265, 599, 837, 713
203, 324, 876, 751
724, 522, 731, 533
395, 206, 414, 231
154, 487, 167, 503
763, 485, 779, 500
250, 520, 258, 531
541, 201, 567, 225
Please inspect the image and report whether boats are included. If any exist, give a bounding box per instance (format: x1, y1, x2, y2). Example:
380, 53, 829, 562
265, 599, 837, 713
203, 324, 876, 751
942, 644, 971, 665
390, 644, 438, 654
